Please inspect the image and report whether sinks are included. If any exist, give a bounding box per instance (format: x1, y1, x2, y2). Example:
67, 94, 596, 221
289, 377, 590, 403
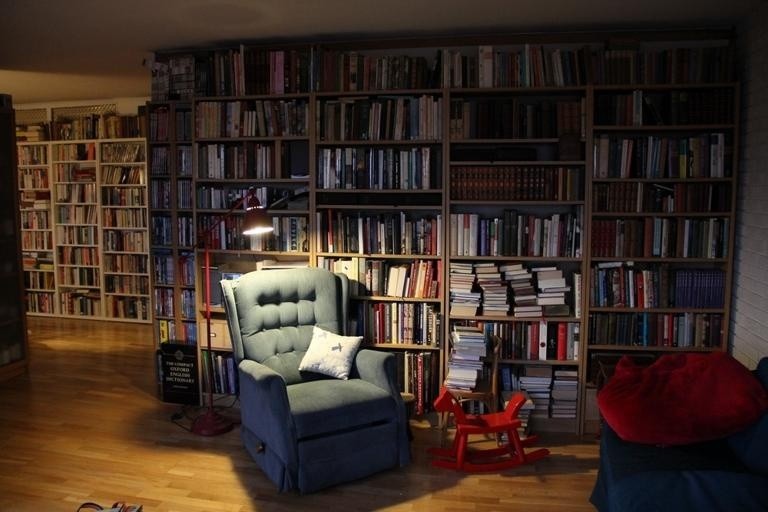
214, 269, 409, 496
588, 358, 766, 512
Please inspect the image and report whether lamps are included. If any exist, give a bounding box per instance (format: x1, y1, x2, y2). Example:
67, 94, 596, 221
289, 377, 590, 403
439, 338, 503, 450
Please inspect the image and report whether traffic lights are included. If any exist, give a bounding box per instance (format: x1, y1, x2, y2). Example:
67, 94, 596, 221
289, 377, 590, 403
300, 331, 361, 381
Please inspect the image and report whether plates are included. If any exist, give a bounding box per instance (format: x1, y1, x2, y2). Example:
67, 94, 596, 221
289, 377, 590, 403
149, 24, 739, 100
97, 138, 152, 323
581, 83, 738, 442
191, 93, 313, 406
16, 141, 57, 318
146, 99, 195, 349
312, 90, 443, 446
0, 94, 29, 382
50, 140, 103, 321
444, 84, 588, 436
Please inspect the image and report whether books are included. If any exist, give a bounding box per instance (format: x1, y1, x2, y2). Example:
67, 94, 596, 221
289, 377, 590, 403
591, 217, 729, 259
194, 101, 311, 179
587, 311, 722, 348
451, 166, 585, 201
449, 205, 584, 258
443, 43, 731, 88
316, 209, 442, 256
148, 109, 195, 342
318, 147, 442, 191
317, 256, 442, 419
198, 188, 309, 251
449, 262, 582, 319
449, 96, 586, 140
17, 105, 149, 320
201, 352, 236, 393
446, 321, 580, 437
593, 181, 731, 213
146, 43, 443, 99
316, 96, 443, 141
593, 131, 732, 178
594, 89, 732, 126
590, 261, 726, 308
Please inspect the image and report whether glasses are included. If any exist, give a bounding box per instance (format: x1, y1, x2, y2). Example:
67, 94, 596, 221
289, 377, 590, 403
179, 184, 272, 436
